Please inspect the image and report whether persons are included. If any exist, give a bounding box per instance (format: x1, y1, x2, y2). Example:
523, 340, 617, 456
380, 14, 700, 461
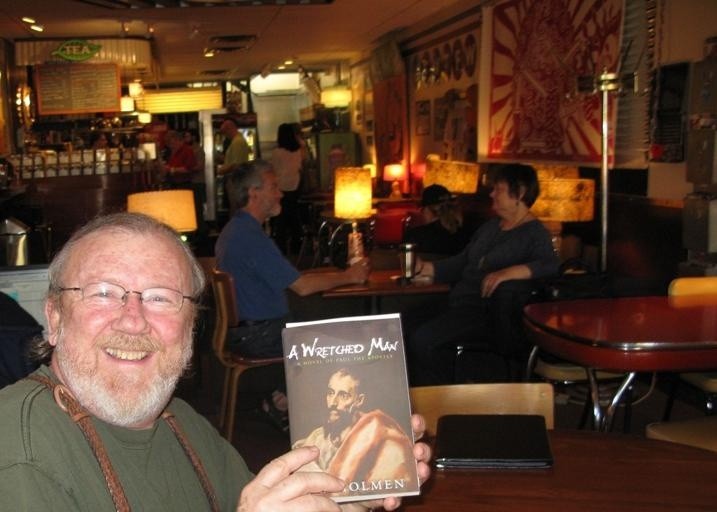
399, 185, 481, 260
290, 369, 421, 498
212, 159, 371, 443
86, 116, 318, 247
0, 210, 434, 512
397, 159, 561, 384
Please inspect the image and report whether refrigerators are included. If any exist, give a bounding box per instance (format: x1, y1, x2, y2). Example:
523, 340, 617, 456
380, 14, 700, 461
211, 112, 259, 222
302, 132, 361, 191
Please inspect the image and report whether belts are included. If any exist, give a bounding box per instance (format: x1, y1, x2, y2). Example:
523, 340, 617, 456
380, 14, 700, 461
238, 320, 265, 327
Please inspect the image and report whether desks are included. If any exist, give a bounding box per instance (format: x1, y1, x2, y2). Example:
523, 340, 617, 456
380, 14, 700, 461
374, 433, 715, 511
525, 292, 716, 432
282, 263, 454, 317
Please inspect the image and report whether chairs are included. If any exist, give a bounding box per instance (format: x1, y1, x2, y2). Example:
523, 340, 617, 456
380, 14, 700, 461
296, 200, 320, 268
644, 414, 716, 454
209, 268, 286, 443
405, 385, 553, 432
525, 342, 636, 434
661, 279, 717, 425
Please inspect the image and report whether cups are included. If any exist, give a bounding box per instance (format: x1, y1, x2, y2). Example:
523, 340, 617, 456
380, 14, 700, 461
396, 243, 425, 278
350, 258, 368, 282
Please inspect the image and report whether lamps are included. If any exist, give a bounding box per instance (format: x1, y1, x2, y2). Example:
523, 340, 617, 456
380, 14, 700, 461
127, 188, 199, 232
382, 164, 407, 197
333, 168, 374, 272
320, 61, 352, 109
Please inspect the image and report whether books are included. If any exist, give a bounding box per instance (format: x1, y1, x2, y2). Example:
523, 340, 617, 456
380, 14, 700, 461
431, 412, 554, 471
279, 311, 423, 504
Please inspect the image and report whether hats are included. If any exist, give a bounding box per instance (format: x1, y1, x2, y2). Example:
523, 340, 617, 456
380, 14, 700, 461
416, 185, 459, 206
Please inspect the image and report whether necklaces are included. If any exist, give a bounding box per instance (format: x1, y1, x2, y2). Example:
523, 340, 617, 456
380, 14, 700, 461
26, 372, 220, 512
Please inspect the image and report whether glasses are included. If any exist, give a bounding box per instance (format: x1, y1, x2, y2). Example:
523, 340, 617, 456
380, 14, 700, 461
59, 282, 196, 315
